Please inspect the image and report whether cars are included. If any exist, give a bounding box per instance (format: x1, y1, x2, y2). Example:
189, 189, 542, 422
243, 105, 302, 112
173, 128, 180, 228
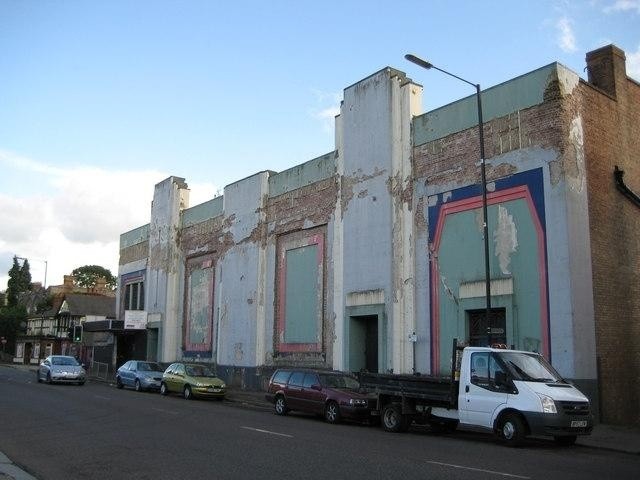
37, 355, 86, 384
267, 369, 377, 422
160, 362, 227, 400
116, 360, 165, 392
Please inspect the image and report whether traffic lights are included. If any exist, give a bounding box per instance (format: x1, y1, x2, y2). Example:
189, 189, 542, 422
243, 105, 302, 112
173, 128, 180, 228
73, 325, 83, 342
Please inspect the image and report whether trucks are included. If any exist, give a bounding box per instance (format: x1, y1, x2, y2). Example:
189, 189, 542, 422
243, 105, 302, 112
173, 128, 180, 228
363, 340, 594, 442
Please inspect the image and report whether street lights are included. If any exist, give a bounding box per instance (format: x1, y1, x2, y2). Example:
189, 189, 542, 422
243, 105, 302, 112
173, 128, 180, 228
405, 54, 492, 346
